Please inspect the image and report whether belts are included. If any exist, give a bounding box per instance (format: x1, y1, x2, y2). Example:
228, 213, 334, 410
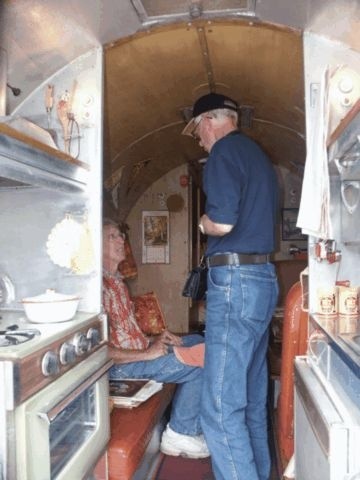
206, 253, 268, 268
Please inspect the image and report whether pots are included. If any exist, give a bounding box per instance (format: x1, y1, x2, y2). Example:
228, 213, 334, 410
16, 289, 85, 323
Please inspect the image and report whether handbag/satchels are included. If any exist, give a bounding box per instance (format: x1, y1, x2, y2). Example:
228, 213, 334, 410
181, 266, 207, 300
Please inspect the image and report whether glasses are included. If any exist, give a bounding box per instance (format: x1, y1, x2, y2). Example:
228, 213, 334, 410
102, 233, 128, 242
191, 115, 213, 140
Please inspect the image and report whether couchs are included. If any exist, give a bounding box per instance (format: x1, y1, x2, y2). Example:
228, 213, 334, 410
90, 332, 189, 480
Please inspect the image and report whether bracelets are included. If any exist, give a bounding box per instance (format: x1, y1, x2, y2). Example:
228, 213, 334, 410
198, 221, 204, 234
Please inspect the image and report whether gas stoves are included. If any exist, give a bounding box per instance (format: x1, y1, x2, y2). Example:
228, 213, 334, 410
0, 309, 110, 412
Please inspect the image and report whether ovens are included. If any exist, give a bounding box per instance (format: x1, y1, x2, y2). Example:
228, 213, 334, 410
8, 344, 112, 480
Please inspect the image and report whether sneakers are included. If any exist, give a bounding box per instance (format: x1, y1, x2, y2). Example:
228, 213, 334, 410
160, 423, 210, 458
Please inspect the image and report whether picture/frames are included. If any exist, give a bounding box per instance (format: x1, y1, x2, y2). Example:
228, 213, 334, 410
280, 208, 308, 241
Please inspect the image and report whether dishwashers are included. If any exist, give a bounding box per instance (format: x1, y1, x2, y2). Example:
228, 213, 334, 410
293, 355, 360, 480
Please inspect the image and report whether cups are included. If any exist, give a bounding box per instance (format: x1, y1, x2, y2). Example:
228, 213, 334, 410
319, 295, 335, 314
335, 286, 360, 316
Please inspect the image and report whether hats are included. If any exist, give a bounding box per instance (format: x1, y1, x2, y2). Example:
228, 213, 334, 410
182, 93, 239, 137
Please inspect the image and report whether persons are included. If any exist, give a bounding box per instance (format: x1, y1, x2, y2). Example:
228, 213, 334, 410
182, 91, 279, 480
102, 220, 211, 459
